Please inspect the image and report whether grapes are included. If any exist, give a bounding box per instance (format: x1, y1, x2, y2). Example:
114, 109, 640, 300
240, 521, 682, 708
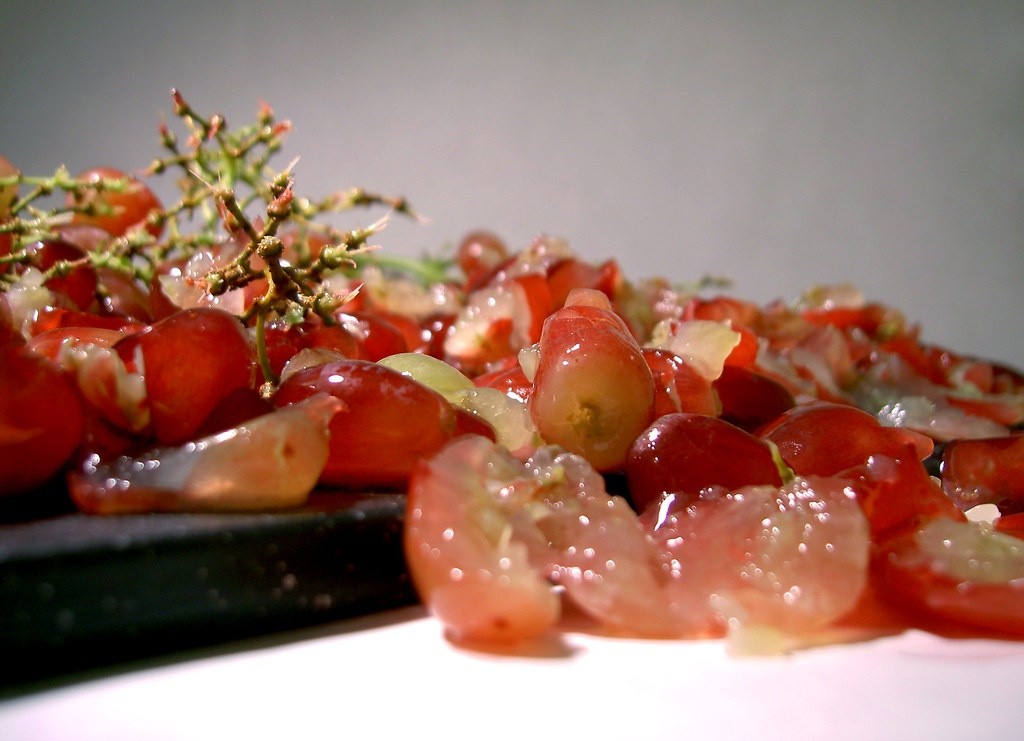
0, 157, 1024, 652
